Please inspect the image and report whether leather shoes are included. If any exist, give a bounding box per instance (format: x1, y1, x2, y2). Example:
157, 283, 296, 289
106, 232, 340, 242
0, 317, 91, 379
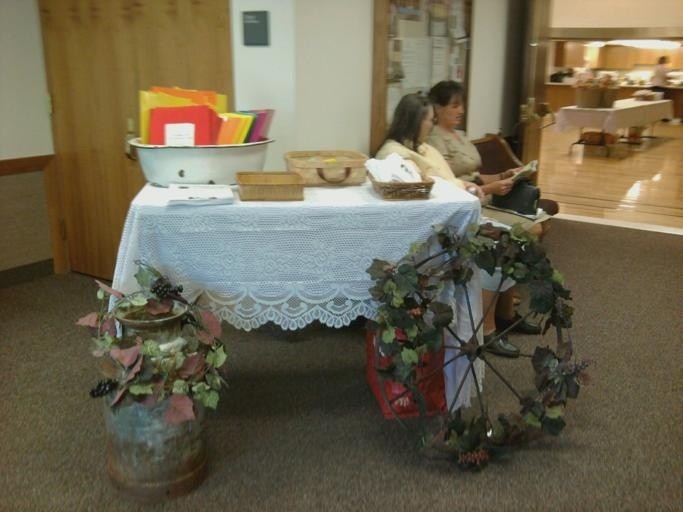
495, 309, 543, 336
481, 328, 521, 358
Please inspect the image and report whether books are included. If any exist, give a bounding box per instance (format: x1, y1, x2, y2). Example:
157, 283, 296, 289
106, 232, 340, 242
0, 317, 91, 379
139, 83, 274, 147
167, 182, 234, 206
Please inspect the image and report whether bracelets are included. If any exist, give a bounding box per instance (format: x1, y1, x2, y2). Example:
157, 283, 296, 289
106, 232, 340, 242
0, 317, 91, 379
500, 172, 505, 179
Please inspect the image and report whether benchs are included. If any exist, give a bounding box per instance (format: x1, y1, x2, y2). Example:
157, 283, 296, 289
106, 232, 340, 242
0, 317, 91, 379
469, 133, 559, 252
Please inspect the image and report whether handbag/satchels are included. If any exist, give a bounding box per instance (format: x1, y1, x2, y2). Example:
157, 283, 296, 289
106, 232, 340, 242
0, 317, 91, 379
491, 176, 541, 218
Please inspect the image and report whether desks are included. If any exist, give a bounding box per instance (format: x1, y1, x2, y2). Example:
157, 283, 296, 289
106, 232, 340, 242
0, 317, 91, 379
108, 176, 480, 427
561, 97, 673, 158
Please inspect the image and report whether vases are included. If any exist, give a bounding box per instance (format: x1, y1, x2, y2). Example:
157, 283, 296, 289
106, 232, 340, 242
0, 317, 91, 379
104, 300, 211, 500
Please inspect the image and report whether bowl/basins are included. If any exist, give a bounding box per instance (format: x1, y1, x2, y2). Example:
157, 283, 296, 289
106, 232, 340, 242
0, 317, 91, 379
128, 135, 278, 188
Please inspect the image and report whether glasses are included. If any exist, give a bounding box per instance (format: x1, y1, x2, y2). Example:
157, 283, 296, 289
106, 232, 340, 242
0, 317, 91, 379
421, 115, 439, 125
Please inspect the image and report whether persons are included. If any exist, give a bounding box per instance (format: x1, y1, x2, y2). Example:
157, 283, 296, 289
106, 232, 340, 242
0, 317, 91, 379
375, 89, 542, 357
428, 79, 543, 306
650, 55, 675, 122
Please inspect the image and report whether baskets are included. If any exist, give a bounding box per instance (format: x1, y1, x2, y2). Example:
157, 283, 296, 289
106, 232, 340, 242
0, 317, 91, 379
234, 170, 306, 203
366, 167, 436, 200
